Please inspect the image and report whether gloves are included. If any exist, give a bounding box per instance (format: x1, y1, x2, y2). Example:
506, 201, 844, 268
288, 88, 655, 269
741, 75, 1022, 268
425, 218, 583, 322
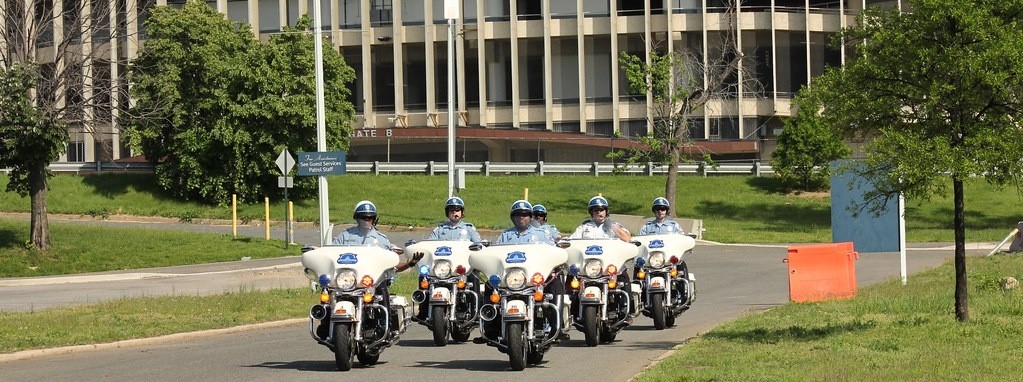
406, 252, 425, 268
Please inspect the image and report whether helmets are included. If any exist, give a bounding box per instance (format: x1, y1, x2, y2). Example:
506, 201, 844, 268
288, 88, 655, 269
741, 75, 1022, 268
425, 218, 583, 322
511, 200, 533, 217
531, 204, 547, 218
588, 196, 609, 214
444, 197, 465, 215
353, 200, 378, 219
651, 197, 669, 214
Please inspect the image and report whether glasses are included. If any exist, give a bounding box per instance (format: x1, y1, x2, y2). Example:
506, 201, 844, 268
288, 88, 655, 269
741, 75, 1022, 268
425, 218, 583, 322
359, 216, 373, 221
448, 207, 461, 212
653, 205, 667, 212
591, 206, 606, 212
534, 212, 544, 217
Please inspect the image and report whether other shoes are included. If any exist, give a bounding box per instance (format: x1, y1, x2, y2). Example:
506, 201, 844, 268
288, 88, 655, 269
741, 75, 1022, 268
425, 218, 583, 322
411, 314, 429, 322
680, 306, 689, 311
317, 325, 327, 340
614, 319, 628, 325
473, 331, 501, 343
548, 327, 570, 341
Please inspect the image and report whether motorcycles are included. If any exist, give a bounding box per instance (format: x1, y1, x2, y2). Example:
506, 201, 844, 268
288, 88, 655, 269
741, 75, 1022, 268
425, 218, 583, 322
627, 232, 698, 330
467, 240, 572, 372
404, 239, 484, 347
297, 242, 412, 371
558, 235, 643, 348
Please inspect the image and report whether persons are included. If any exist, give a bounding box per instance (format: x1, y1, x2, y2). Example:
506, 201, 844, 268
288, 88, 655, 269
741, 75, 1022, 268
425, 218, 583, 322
473, 200, 571, 344
316, 200, 424, 342
411, 197, 482, 325
564, 195, 636, 327
635, 197, 696, 310
